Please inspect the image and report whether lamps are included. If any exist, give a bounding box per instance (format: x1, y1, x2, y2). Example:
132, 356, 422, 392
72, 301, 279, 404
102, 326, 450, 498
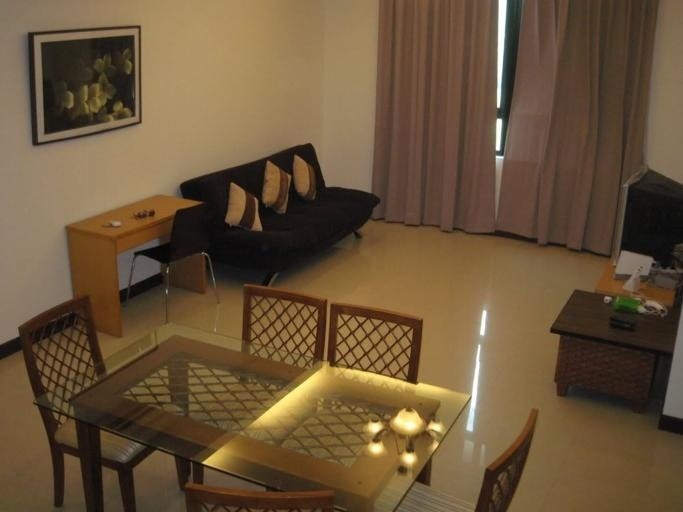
361, 406, 445, 479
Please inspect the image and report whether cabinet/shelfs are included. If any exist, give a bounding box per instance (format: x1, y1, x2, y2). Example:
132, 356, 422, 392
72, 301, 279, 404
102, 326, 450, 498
594, 259, 680, 308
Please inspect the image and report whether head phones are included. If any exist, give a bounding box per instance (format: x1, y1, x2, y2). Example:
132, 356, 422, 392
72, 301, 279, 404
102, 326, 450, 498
133, 206, 156, 219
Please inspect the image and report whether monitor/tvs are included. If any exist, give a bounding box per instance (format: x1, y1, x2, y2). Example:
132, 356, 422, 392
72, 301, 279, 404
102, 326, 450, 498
613, 163, 683, 268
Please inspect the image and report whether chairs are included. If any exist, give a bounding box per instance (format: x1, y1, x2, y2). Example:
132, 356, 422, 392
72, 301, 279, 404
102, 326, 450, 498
279, 302, 423, 468
118, 197, 222, 324
368, 406, 541, 511
192, 282, 328, 493
12, 292, 188, 512
178, 475, 335, 511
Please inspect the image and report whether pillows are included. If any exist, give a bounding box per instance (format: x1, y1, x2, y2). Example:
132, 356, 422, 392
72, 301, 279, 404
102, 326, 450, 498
290, 151, 319, 203
221, 181, 266, 232
259, 158, 294, 216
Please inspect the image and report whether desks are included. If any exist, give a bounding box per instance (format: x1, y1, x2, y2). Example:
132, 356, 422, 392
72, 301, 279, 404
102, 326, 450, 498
28, 317, 475, 511
544, 285, 677, 416
61, 194, 210, 340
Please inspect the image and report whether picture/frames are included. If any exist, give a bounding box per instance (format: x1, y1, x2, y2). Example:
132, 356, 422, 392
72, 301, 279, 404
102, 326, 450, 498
24, 23, 144, 150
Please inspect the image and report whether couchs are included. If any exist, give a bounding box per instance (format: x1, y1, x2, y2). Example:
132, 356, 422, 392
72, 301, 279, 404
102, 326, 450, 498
179, 140, 382, 287
